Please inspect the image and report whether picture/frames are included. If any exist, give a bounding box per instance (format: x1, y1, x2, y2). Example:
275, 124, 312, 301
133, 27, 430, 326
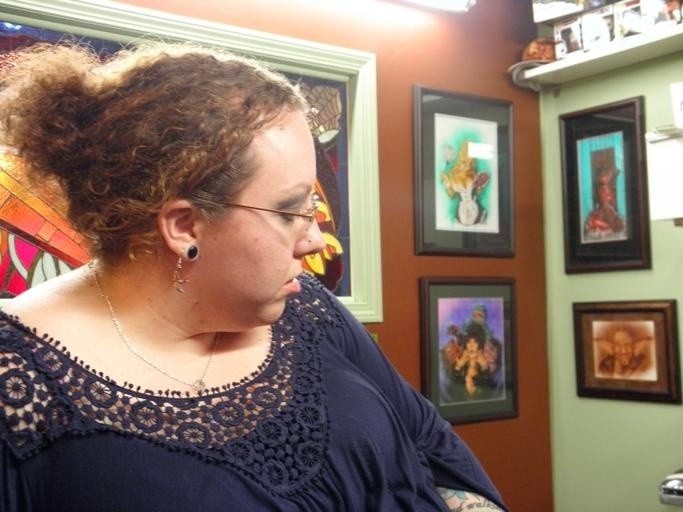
569, 299, 681, 407
415, 274, 521, 425
0, 0, 388, 334
555, 95, 655, 276
408, 78, 519, 261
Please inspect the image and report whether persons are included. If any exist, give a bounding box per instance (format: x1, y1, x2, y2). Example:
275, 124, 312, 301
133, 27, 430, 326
0, 27, 507, 512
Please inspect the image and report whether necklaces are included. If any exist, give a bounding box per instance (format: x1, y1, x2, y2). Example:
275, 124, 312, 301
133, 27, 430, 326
84, 260, 223, 393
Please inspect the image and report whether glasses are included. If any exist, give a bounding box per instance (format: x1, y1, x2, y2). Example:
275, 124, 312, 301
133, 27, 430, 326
223, 201, 320, 223
